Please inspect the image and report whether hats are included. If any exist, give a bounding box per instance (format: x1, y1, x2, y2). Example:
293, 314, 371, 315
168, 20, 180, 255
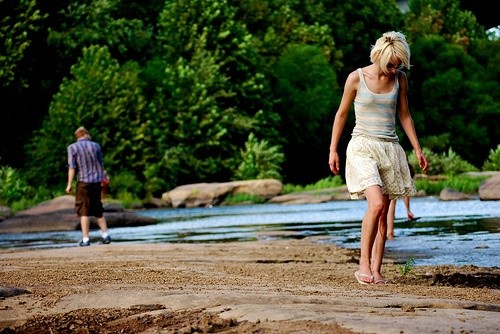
74, 128, 88, 138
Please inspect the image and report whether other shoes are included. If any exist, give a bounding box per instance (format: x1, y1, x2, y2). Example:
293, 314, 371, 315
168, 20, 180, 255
79, 240, 90, 246
102, 236, 111, 244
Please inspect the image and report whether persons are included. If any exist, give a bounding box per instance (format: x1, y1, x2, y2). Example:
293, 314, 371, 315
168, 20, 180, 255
324, 32, 430, 288
385, 166, 418, 240
64, 127, 113, 246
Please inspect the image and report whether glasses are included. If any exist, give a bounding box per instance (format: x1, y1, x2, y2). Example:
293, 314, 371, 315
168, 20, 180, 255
387, 63, 401, 69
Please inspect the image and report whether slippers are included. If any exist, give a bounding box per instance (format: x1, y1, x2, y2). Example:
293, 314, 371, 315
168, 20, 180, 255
373, 278, 388, 286
355, 271, 374, 285
